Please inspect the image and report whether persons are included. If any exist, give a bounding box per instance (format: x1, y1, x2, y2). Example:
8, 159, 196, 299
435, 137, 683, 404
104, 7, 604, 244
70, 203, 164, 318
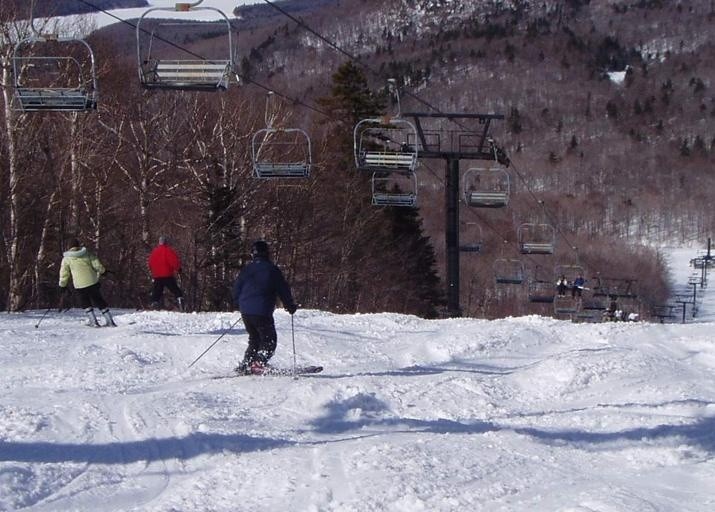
57, 239, 116, 328
572, 274, 584, 297
556, 273, 566, 298
147, 236, 189, 313
233, 242, 295, 378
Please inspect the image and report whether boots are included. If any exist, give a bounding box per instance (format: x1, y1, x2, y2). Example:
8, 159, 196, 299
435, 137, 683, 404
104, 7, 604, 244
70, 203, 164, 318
101, 307, 117, 327
84, 306, 100, 327
234, 349, 274, 376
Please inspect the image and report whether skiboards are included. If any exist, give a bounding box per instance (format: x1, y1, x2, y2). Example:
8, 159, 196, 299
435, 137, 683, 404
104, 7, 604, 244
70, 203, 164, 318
211, 365, 323, 379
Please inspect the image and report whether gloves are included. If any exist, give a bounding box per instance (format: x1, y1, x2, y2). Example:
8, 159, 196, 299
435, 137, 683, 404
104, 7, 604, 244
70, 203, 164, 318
284, 304, 298, 314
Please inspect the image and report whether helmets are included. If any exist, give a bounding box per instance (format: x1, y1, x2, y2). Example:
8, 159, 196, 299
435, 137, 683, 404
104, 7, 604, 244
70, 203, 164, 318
67, 237, 80, 250
249, 241, 270, 259
